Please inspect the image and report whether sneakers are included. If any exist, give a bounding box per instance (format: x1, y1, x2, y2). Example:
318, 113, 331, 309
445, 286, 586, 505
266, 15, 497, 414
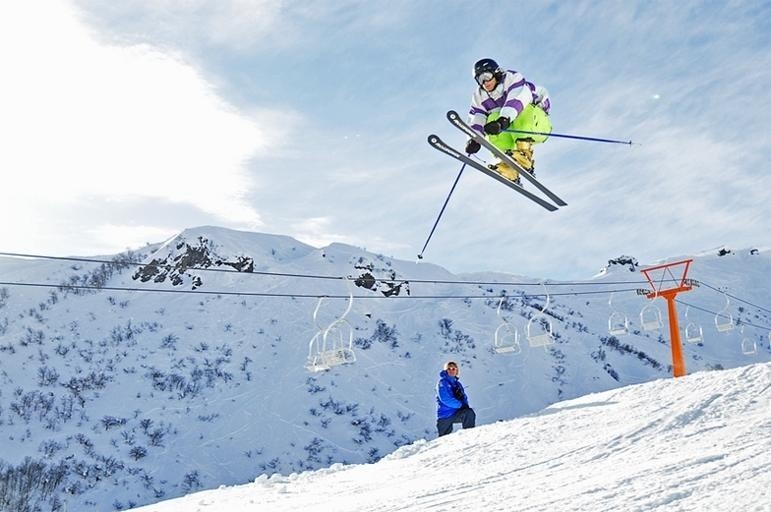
489, 149, 534, 182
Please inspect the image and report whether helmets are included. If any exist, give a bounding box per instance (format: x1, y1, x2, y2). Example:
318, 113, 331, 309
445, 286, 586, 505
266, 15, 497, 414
473, 59, 502, 87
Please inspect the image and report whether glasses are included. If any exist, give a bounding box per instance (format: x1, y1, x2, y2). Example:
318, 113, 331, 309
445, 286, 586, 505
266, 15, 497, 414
478, 72, 494, 85
448, 367, 456, 371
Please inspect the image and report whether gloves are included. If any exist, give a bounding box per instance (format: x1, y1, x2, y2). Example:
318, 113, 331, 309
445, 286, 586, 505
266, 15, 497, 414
466, 140, 481, 154
484, 121, 503, 134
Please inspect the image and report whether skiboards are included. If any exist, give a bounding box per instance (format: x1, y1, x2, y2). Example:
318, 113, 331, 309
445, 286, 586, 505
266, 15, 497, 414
427, 110, 567, 212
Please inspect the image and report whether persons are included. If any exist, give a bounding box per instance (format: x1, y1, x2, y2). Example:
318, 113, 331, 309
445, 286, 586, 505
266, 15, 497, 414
460, 57, 553, 187
435, 359, 476, 439
